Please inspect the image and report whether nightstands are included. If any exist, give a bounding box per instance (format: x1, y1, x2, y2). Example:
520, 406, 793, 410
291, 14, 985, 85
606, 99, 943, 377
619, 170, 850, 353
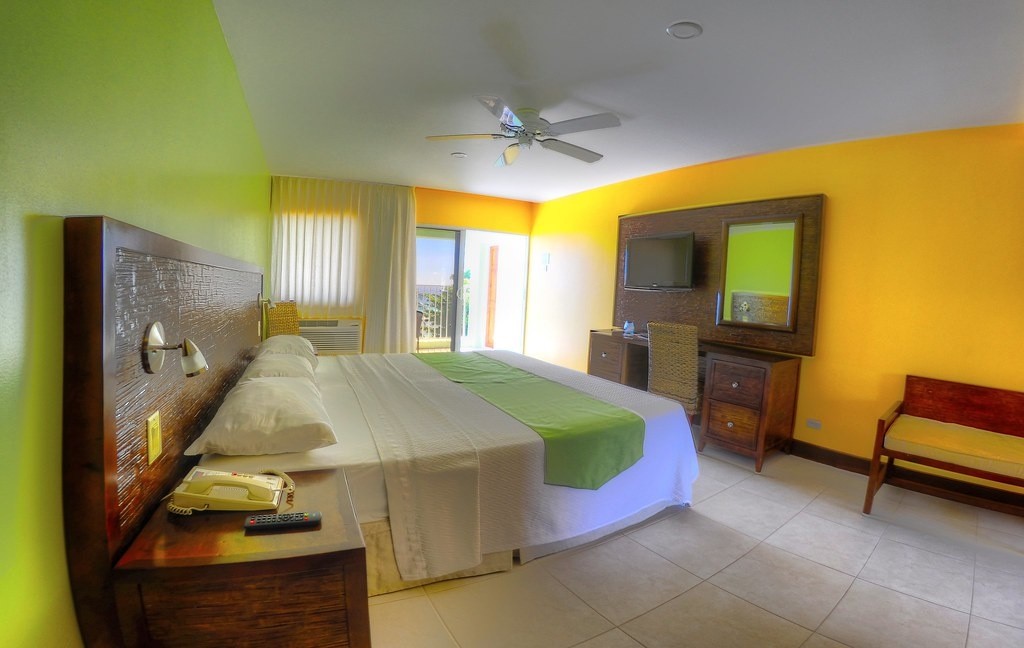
114, 466, 372, 648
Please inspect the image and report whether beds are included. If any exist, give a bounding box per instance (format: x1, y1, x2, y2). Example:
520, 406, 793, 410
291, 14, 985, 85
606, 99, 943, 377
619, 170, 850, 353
192, 350, 698, 598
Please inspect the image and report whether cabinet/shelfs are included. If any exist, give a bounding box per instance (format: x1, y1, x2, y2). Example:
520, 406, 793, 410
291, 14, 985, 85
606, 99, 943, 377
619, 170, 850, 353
588, 327, 801, 473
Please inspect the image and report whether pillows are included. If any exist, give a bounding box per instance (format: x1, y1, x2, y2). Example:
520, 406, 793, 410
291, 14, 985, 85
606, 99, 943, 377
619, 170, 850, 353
236, 354, 317, 385
184, 377, 338, 455
254, 335, 319, 371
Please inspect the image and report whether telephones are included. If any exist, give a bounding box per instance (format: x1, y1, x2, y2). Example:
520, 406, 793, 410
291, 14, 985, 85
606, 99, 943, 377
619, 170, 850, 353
174, 465, 284, 511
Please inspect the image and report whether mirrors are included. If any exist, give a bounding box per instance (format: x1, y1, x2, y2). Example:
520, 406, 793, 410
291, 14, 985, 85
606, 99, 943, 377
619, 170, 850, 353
715, 212, 804, 332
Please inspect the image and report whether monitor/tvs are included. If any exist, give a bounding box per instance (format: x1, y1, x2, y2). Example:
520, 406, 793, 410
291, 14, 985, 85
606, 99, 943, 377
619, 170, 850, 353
624, 231, 695, 292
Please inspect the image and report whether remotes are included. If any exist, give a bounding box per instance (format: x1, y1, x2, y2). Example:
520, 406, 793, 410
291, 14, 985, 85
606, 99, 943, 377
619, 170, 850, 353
242, 509, 322, 530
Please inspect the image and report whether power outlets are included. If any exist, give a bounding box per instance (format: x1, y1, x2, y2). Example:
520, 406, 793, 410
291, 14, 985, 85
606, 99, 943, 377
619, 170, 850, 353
806, 418, 822, 430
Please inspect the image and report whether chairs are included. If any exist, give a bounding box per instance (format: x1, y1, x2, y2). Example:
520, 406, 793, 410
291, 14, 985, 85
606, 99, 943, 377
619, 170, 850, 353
647, 320, 705, 427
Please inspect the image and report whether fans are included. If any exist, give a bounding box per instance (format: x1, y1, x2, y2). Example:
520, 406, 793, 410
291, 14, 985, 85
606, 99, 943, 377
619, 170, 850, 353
424, 96, 622, 166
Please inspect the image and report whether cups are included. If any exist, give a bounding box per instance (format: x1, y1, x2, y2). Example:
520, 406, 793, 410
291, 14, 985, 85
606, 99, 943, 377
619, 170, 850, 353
623, 319, 635, 333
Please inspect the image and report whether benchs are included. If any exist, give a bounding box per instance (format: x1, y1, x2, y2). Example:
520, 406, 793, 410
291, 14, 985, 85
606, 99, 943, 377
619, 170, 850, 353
863, 374, 1024, 515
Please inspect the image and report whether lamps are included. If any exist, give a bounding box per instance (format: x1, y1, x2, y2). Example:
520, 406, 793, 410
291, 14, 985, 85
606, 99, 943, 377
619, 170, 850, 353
257, 293, 277, 310
265, 300, 300, 338
142, 321, 209, 378
